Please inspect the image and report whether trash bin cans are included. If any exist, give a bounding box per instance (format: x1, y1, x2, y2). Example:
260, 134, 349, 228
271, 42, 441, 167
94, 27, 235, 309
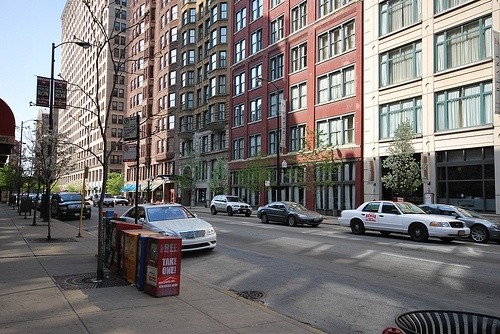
395, 310, 500, 334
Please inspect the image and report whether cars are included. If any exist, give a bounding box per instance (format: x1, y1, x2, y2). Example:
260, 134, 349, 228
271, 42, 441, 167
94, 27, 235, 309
337, 198, 471, 243
257, 200, 323, 228
11, 191, 129, 222
416, 202, 500, 244
119, 202, 217, 255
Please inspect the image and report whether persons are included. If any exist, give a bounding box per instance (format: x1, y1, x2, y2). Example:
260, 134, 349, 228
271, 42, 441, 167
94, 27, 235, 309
137, 209, 150, 221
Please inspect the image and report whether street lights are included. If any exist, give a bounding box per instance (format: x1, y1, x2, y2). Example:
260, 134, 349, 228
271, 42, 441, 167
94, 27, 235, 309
139, 130, 150, 203
41, 41, 91, 222
16, 119, 39, 207
257, 77, 280, 202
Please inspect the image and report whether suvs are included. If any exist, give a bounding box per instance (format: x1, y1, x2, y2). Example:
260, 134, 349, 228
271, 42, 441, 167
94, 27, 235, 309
209, 194, 252, 217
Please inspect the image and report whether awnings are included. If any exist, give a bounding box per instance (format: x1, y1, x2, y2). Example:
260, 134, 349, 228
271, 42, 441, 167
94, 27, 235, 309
119, 183, 148, 192
143, 179, 163, 191
0, 98, 15, 168
